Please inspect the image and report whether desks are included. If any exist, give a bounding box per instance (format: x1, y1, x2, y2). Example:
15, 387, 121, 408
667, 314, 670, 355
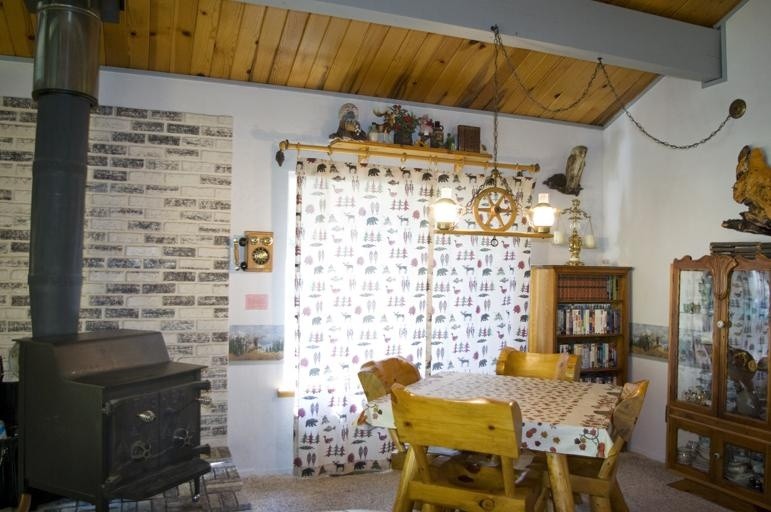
363, 370, 623, 511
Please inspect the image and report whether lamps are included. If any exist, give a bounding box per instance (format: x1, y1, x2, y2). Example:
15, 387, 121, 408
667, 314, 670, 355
427, 168, 558, 239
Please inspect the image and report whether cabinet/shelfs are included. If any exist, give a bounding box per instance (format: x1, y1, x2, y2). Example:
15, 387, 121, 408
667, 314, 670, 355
529, 266, 633, 451
667, 251, 770, 512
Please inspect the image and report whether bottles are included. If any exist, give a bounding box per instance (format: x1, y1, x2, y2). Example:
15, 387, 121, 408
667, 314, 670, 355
368, 122, 378, 143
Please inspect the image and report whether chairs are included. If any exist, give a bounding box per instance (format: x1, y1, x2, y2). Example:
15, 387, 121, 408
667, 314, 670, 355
496, 346, 581, 382
358, 357, 435, 512
541, 380, 648, 511
391, 382, 547, 512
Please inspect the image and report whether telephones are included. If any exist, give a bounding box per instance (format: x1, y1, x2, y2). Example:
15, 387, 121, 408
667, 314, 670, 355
232, 231, 273, 273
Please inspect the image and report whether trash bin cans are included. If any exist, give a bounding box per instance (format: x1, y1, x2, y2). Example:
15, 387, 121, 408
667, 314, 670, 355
0, 425, 18, 508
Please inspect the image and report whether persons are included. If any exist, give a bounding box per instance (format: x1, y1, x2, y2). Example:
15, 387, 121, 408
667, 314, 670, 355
329, 102, 368, 142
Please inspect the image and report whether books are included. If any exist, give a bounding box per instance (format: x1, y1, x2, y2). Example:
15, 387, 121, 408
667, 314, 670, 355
556, 273, 620, 384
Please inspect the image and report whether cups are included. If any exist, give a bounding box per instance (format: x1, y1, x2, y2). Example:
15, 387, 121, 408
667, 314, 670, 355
678, 448, 692, 466
